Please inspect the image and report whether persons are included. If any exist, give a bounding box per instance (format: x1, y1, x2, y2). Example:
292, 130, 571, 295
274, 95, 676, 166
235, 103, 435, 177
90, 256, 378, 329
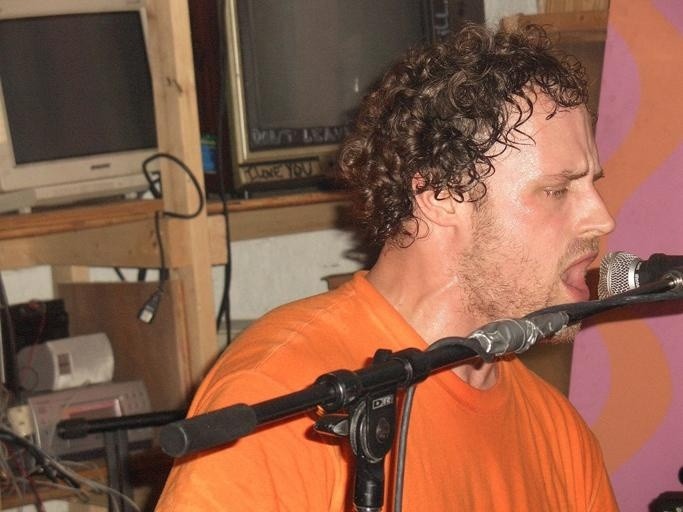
153, 16, 619, 512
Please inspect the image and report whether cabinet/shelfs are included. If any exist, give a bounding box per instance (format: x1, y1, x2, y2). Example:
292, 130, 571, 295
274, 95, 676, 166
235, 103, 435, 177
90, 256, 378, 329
1, 0, 498, 512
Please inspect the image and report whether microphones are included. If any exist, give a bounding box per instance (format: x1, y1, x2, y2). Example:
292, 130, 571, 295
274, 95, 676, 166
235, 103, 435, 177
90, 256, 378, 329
597, 250, 683, 306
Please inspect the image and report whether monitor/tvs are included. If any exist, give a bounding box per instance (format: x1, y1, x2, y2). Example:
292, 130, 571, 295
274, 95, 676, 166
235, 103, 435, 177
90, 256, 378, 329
222, 0, 450, 202
0, 0, 166, 212
